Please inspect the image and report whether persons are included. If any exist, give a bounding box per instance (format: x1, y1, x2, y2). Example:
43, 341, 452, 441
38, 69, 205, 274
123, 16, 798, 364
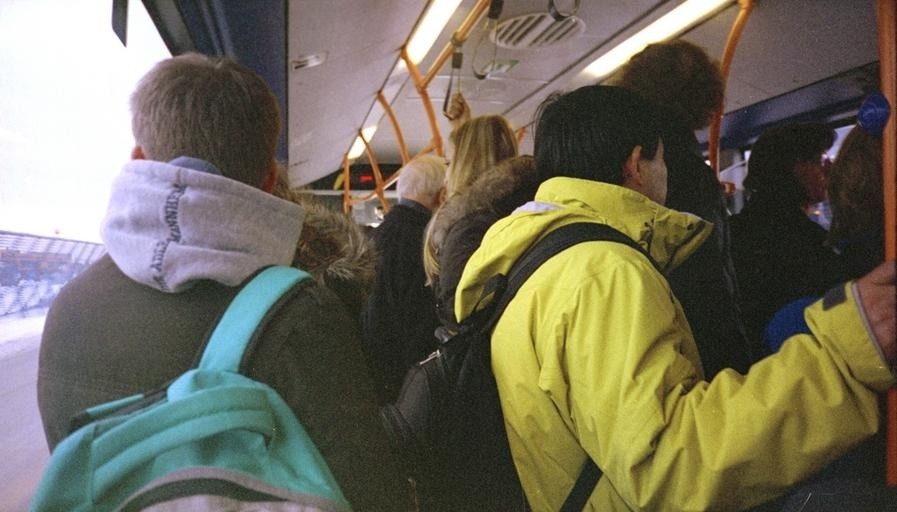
35, 41, 897, 511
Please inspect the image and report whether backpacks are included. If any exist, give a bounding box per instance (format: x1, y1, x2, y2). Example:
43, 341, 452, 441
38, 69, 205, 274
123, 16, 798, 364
31, 265, 352, 512
379, 221, 662, 512
